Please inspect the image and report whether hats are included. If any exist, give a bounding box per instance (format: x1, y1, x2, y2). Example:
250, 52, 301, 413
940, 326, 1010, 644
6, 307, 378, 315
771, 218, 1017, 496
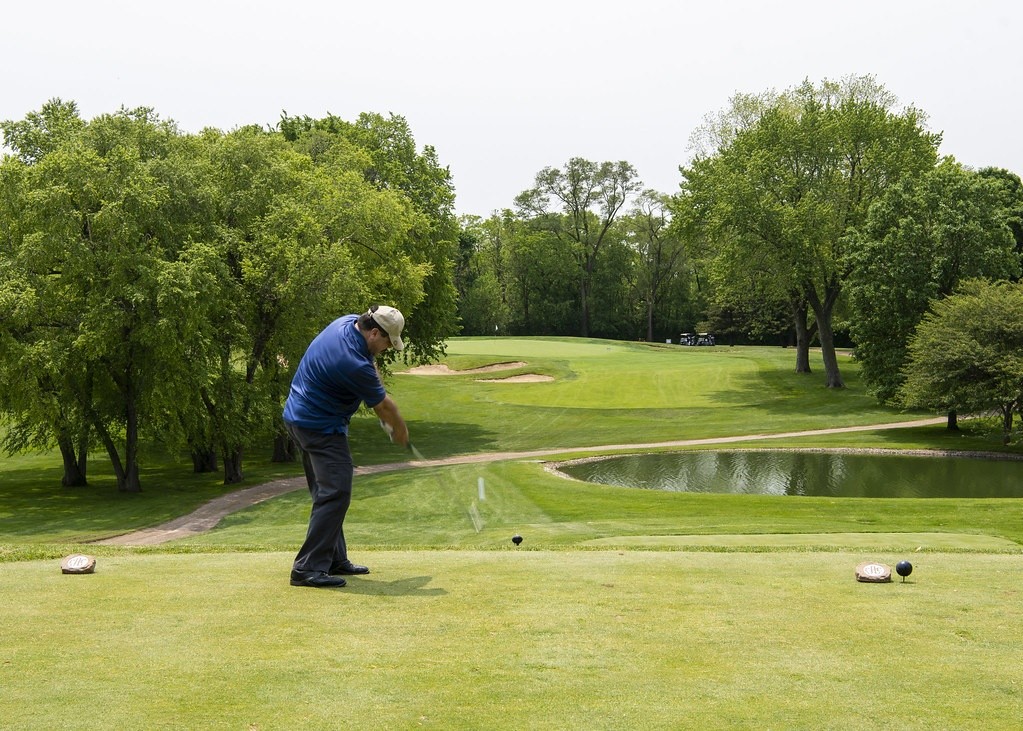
367, 306, 404, 350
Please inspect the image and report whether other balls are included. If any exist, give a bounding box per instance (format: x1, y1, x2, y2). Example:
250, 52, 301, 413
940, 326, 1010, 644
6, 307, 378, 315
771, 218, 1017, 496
512, 535, 524, 544
896, 560, 913, 577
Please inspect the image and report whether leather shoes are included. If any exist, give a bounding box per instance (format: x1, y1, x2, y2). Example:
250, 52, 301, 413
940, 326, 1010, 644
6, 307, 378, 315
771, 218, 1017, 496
290, 569, 346, 588
327, 561, 369, 575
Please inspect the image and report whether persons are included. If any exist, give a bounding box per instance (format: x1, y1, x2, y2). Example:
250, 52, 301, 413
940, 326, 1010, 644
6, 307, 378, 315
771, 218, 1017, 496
283, 306, 409, 586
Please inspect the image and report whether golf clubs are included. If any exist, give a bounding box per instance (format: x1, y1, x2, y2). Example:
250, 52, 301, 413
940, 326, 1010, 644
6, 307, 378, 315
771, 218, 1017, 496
378, 419, 485, 535
